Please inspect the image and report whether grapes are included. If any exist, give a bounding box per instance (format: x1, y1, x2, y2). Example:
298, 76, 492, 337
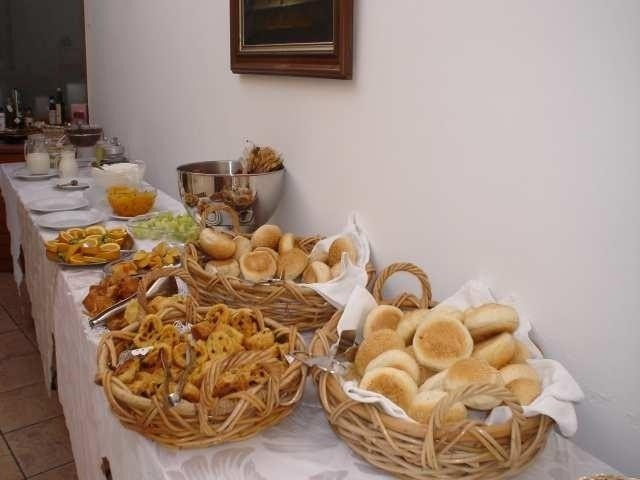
129, 211, 198, 240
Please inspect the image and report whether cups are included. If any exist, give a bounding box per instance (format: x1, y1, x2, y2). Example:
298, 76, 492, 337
54, 151, 79, 178
96, 147, 104, 160
23, 134, 50, 175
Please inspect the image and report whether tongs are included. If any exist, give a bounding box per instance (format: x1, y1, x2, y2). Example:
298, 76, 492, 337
89, 271, 178, 330
275, 329, 358, 374
115, 320, 193, 408
258, 270, 285, 284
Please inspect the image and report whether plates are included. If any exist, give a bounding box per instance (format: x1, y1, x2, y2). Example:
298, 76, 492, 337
14, 167, 161, 267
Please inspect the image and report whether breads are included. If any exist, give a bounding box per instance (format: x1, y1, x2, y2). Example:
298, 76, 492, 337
345, 302, 543, 428
195, 225, 358, 285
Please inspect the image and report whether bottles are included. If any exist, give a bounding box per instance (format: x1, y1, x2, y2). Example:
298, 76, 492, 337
14, 88, 24, 129
56, 87, 65, 125
5, 96, 16, 128
0, 112, 6, 131
24, 107, 34, 128
48, 95, 56, 125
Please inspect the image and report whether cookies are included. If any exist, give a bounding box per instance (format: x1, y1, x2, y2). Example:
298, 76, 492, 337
82, 275, 139, 317
115, 303, 288, 403
111, 242, 185, 278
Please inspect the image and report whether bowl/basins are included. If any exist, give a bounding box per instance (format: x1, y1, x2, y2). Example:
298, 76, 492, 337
87, 158, 146, 192
126, 211, 202, 255
65, 129, 102, 146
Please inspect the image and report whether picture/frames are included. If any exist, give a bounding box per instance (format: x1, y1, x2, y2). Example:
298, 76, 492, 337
228, 0, 354, 79
